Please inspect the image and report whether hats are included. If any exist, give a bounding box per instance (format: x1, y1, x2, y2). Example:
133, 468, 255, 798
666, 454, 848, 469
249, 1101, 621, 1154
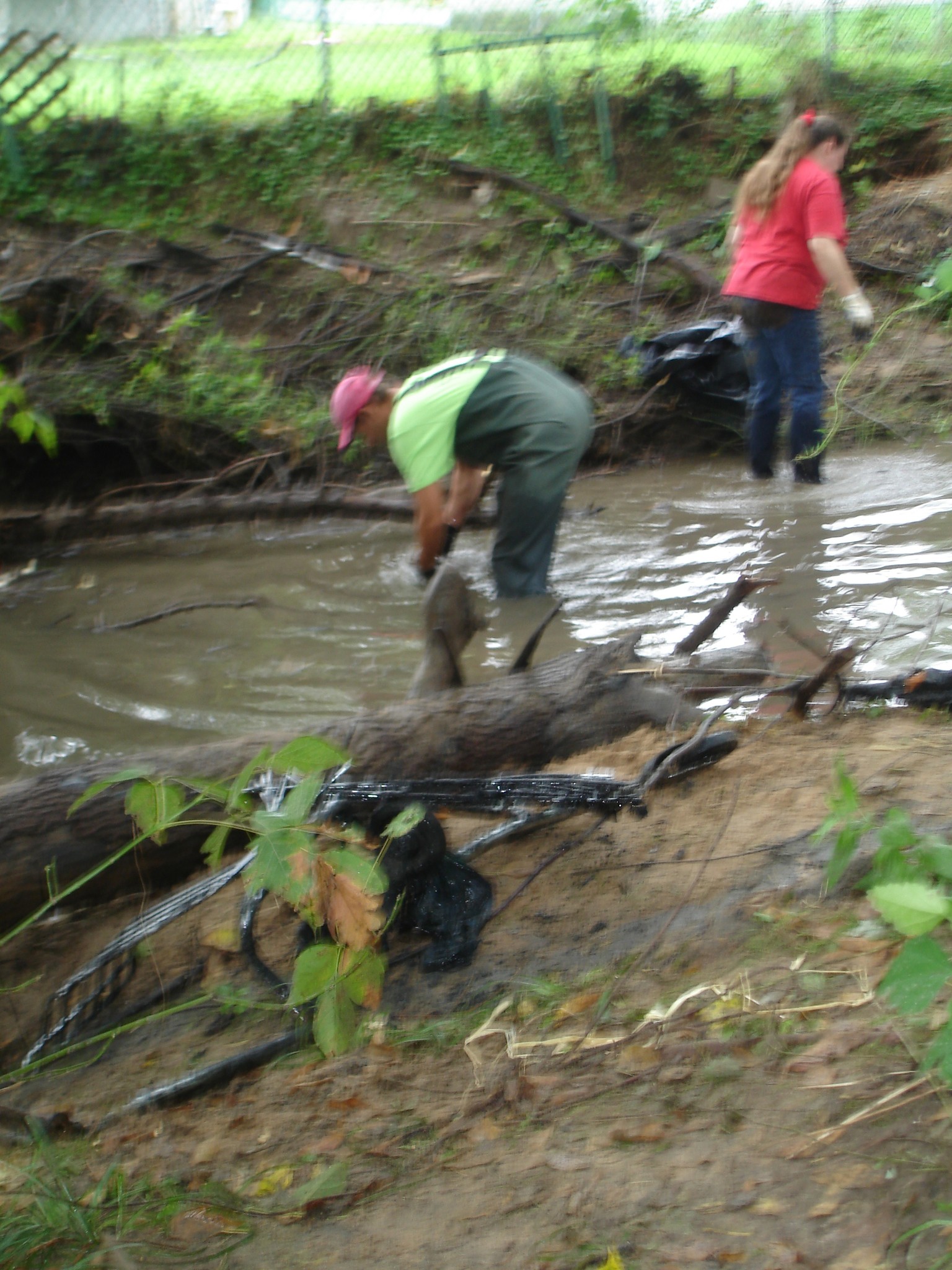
330, 364, 385, 450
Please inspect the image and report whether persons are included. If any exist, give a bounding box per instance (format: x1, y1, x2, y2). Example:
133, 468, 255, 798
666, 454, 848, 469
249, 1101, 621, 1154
330, 348, 595, 598
721, 111, 874, 481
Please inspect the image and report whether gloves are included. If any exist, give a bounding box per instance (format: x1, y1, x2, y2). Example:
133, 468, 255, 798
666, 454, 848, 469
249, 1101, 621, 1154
421, 567, 436, 581
439, 522, 458, 555
841, 292, 875, 339
